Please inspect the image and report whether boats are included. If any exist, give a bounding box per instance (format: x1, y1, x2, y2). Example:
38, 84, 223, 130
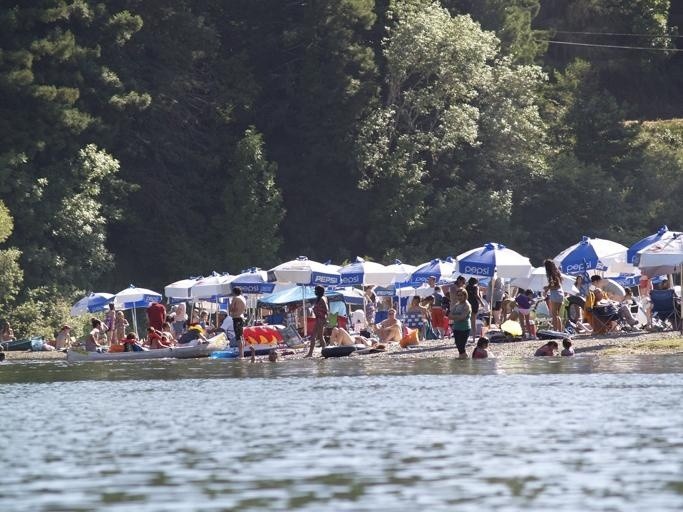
66, 341, 175, 361
0, 334, 46, 351
239, 323, 286, 344
137, 332, 231, 360
205, 335, 312, 359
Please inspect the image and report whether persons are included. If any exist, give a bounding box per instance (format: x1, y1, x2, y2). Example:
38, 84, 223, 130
40, 339, 56, 351
143, 287, 248, 361
56, 324, 72, 348
0, 322, 13, 343
449, 259, 681, 358
258, 275, 447, 360
88, 304, 136, 353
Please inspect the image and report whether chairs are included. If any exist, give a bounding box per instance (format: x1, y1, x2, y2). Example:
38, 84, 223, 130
274, 321, 312, 355
586, 307, 629, 334
648, 289, 682, 331
